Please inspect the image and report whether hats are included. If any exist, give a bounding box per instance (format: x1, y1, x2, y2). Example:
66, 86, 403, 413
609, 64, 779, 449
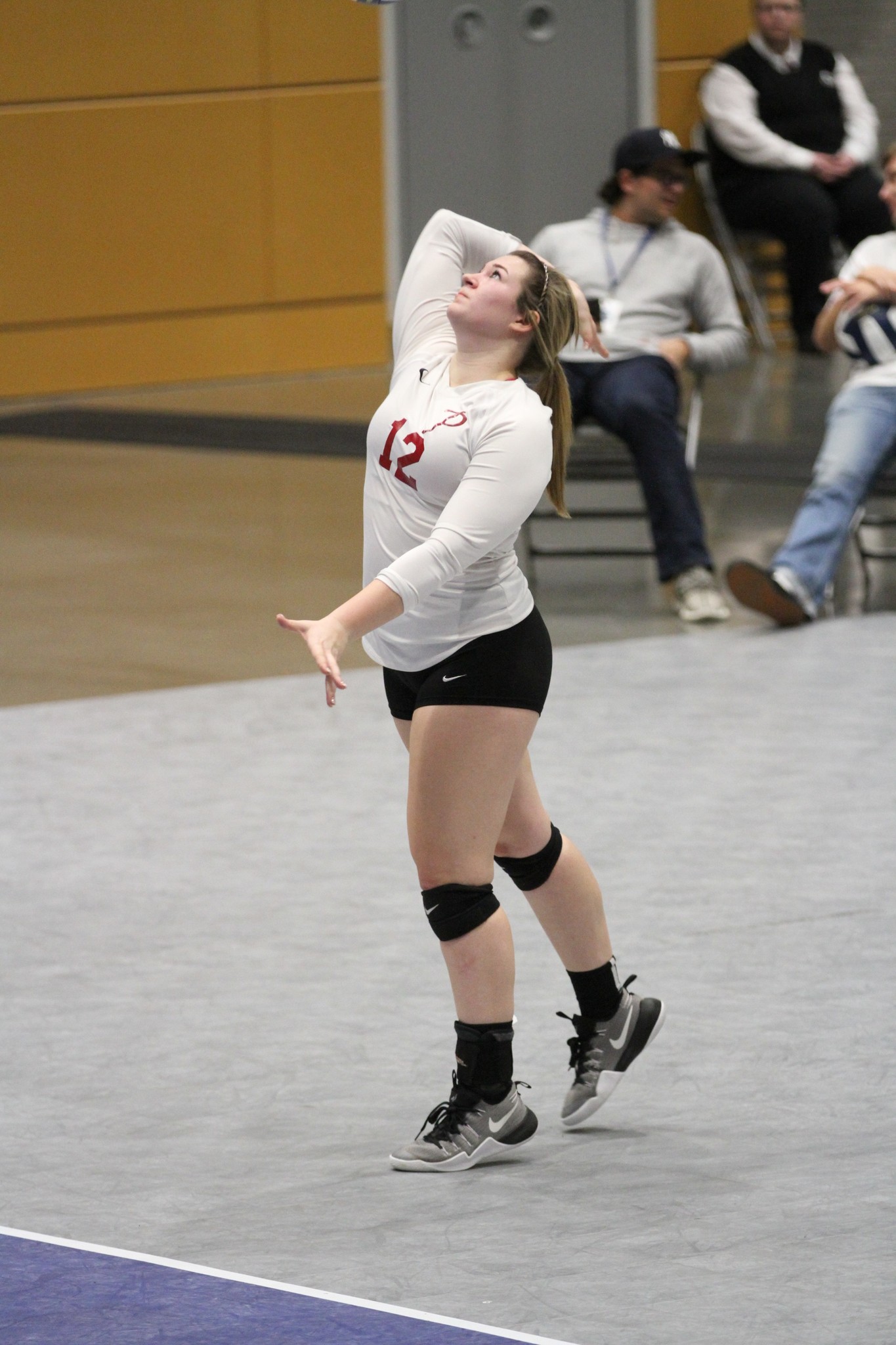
613, 126, 710, 170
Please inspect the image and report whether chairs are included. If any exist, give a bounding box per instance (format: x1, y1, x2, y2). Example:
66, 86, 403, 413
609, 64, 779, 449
686, 117, 848, 355
509, 361, 706, 599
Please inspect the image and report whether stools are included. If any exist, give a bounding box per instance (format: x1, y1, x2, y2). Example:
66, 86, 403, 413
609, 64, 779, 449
844, 455, 896, 610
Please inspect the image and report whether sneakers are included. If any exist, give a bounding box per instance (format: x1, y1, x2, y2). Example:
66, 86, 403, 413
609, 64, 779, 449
389, 1070, 540, 1171
557, 974, 666, 1126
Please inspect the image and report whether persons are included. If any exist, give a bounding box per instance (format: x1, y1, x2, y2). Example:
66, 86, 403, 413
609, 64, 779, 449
274, 209, 668, 1172
696, 0, 896, 357
723, 141, 896, 622
523, 126, 751, 623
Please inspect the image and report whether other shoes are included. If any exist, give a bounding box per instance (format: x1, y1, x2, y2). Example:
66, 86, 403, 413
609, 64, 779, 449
726, 556, 817, 625
673, 565, 731, 622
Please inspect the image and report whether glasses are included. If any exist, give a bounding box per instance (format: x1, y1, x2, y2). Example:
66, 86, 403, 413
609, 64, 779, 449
646, 167, 696, 188
